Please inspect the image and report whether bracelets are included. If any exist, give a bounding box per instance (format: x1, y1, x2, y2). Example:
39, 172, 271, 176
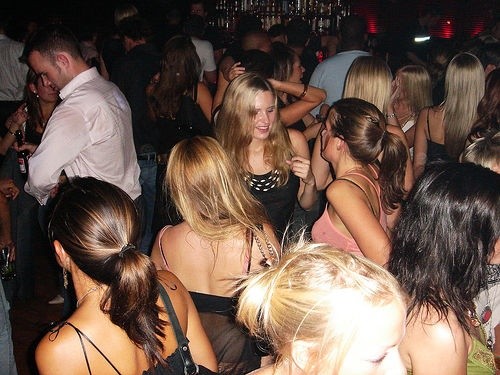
300, 84, 307, 98
387, 113, 395, 118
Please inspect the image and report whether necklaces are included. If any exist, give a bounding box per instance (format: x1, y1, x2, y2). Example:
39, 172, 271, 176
481, 286, 492, 325
35, 114, 48, 127
481, 259, 498, 351
7, 128, 15, 135
442, 299, 480, 327
398, 112, 410, 121
77, 283, 107, 308
345, 167, 362, 174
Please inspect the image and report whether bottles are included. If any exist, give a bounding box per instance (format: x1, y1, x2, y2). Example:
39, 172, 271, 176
209, 0, 351, 36
15, 129, 31, 177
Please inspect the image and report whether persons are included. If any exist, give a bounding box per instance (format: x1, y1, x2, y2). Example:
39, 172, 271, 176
0, 0, 500, 375
